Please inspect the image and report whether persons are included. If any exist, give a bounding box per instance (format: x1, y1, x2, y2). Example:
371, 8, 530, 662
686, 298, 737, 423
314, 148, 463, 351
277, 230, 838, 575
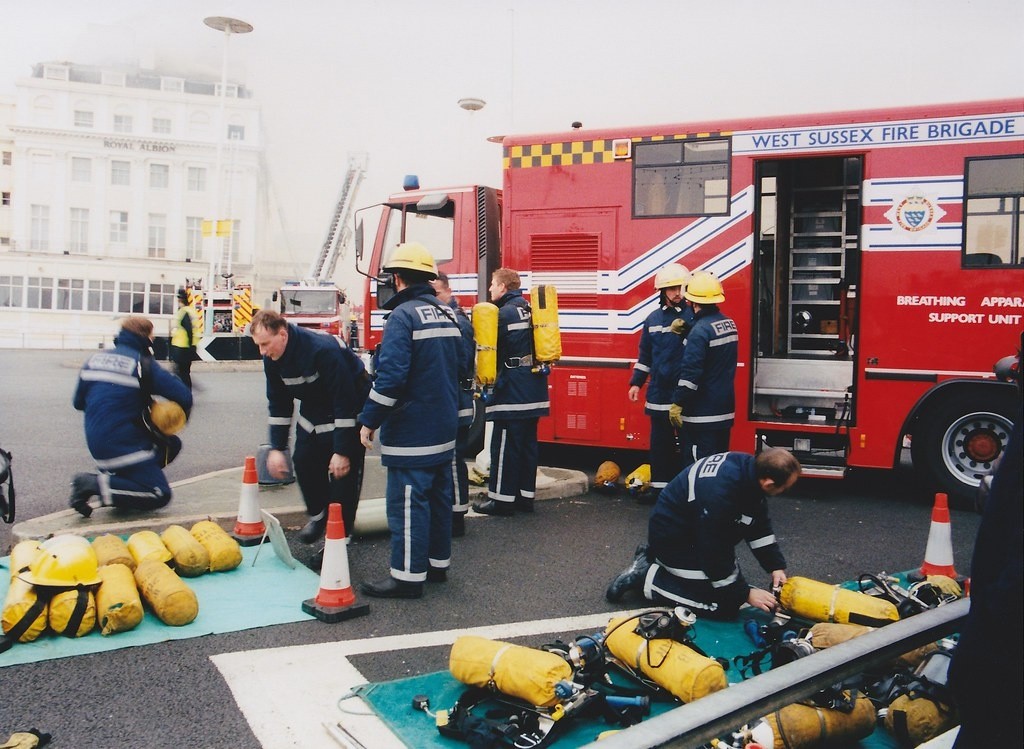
471, 267, 550, 516
606, 446, 802, 620
669, 270, 739, 468
67, 315, 192, 518
168, 288, 201, 397
359, 244, 469, 598
427, 271, 478, 537
250, 306, 373, 548
629, 263, 695, 500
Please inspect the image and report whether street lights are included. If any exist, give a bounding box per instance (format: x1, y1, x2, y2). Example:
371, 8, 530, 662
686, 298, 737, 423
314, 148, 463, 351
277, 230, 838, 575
202, 14, 254, 291
457, 97, 486, 185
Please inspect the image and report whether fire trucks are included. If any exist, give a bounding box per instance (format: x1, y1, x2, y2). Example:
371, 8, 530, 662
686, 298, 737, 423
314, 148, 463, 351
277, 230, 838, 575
184, 273, 252, 338
354, 97, 1024, 512
271, 280, 351, 342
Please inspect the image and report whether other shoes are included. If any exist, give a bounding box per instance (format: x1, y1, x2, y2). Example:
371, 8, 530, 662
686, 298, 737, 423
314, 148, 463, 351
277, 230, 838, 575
452, 513, 465, 537
70, 471, 99, 517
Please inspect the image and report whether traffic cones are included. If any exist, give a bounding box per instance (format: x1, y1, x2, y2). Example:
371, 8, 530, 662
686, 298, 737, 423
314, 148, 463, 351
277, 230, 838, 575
907, 492, 966, 591
300, 501, 371, 626
228, 456, 270, 547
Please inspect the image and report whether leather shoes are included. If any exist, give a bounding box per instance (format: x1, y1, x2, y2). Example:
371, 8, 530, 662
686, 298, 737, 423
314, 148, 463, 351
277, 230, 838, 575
472, 499, 515, 516
299, 514, 325, 569
514, 494, 536, 512
358, 571, 447, 597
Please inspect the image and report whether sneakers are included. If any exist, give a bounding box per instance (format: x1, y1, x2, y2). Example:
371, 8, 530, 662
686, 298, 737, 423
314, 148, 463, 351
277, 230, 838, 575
607, 543, 650, 603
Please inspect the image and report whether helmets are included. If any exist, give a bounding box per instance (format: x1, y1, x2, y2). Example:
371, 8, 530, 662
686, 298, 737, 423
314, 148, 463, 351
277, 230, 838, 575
383, 242, 438, 280
654, 261, 690, 292
685, 270, 725, 304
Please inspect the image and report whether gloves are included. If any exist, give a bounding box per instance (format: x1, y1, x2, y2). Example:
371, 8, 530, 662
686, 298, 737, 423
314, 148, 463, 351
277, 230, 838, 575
669, 402, 683, 429
670, 318, 686, 334
